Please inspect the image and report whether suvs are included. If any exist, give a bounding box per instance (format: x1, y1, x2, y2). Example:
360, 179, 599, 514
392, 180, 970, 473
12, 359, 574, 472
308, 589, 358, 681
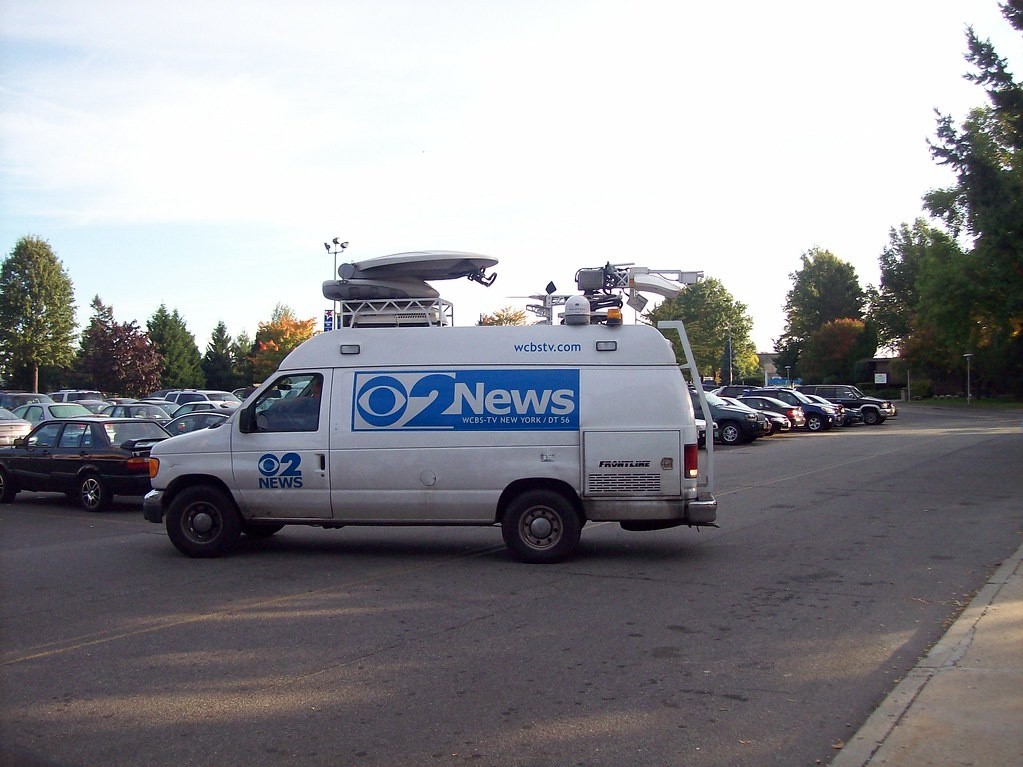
796, 384, 899, 426
688, 384, 773, 446
742, 386, 842, 432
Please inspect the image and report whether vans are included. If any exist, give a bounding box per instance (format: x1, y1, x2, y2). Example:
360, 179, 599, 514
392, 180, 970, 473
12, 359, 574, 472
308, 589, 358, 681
140, 248, 722, 566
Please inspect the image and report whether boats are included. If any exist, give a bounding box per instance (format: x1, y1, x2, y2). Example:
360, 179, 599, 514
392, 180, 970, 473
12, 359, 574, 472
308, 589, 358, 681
322, 278, 441, 307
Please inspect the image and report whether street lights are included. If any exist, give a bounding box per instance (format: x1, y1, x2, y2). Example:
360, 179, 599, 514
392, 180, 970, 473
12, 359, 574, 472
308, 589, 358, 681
785, 365, 791, 385
964, 353, 974, 405
324, 237, 351, 330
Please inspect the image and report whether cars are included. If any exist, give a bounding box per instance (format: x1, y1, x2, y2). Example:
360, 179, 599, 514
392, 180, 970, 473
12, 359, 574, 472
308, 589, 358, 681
11, 402, 117, 443
694, 417, 719, 451
688, 381, 761, 400
0, 406, 39, 446
0, 418, 174, 513
738, 395, 805, 428
137, 406, 237, 448
833, 406, 864, 427
0, 380, 310, 426
805, 391, 846, 423
719, 396, 792, 437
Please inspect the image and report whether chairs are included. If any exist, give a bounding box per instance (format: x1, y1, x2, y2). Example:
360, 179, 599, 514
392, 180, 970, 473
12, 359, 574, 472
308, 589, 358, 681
76, 434, 93, 447
43, 436, 63, 447
136, 411, 147, 418
114, 433, 131, 444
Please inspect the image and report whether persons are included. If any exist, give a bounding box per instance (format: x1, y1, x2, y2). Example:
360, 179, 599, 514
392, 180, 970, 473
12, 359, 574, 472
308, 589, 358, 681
306, 379, 321, 427
